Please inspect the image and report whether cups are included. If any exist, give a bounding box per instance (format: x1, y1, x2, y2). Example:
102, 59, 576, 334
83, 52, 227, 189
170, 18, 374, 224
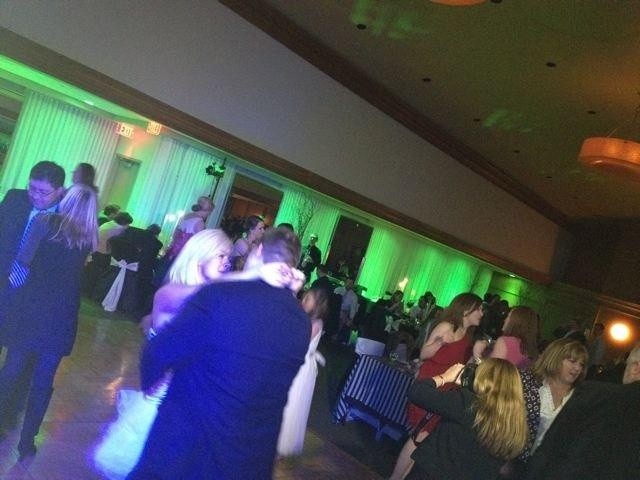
389, 350, 399, 362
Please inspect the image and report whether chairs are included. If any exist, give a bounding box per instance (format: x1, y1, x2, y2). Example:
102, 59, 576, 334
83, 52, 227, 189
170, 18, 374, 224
355, 336, 386, 359
93, 236, 142, 313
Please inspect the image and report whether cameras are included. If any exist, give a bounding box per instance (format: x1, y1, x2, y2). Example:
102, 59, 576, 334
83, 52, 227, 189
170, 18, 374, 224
460, 362, 478, 387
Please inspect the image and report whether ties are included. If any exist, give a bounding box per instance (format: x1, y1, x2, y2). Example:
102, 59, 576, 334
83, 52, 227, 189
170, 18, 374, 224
8, 209, 46, 290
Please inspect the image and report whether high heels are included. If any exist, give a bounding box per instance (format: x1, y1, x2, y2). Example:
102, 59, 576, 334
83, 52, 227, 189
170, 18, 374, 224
17, 440, 36, 461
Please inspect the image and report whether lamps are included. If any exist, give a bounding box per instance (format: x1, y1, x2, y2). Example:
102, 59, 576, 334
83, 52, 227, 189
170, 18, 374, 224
579, 106, 640, 183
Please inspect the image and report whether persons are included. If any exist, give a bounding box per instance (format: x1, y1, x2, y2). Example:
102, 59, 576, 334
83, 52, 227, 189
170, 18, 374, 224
0, 161, 640, 480
